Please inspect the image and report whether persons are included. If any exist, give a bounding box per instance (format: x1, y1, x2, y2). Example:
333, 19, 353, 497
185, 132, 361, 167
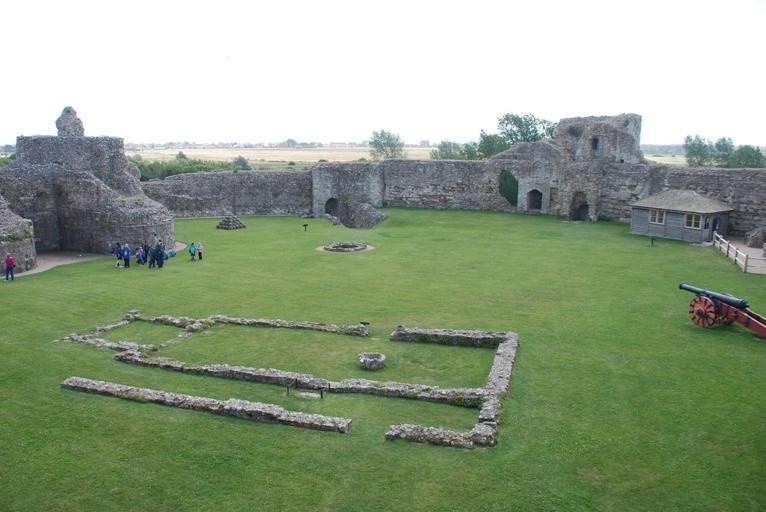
3, 253, 15, 280
113, 233, 203, 271
